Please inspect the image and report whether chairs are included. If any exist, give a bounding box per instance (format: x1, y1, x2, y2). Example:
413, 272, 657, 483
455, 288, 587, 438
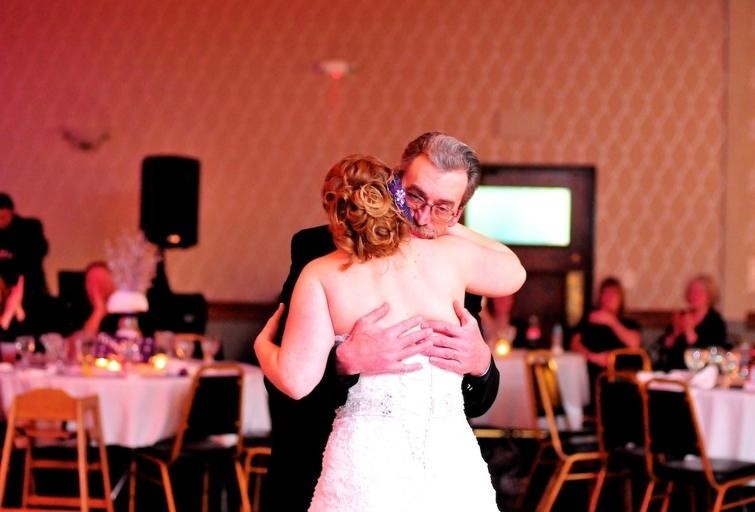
534, 358, 631, 511
516, 355, 606, 510
241, 434, 272, 480
612, 350, 651, 372
1, 388, 114, 511
106, 364, 253, 512
638, 377, 754, 511
598, 372, 699, 511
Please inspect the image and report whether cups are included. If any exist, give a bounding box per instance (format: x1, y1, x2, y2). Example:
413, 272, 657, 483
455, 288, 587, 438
683, 341, 751, 390
0, 328, 220, 379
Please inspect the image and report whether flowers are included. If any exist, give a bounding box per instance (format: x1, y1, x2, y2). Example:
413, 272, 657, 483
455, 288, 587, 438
389, 177, 413, 225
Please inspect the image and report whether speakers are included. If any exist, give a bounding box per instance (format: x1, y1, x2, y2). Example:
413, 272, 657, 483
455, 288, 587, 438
139, 154, 199, 249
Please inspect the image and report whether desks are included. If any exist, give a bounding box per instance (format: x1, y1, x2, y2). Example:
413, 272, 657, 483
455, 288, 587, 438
1, 356, 271, 450
469, 347, 593, 434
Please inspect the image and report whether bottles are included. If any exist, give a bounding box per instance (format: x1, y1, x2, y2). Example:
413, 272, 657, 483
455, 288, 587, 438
562, 252, 585, 328
509, 313, 575, 358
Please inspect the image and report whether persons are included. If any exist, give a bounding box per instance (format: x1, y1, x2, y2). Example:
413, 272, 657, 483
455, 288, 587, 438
63, 261, 124, 340
657, 276, 726, 368
252, 153, 528, 512
258, 131, 499, 512
0, 258, 31, 342
570, 278, 641, 417
0, 194, 64, 359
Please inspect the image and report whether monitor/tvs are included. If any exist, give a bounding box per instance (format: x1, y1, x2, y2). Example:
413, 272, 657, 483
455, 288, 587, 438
462, 185, 573, 250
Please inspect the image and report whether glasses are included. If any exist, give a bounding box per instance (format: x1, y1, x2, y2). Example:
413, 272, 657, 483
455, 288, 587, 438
406, 190, 460, 224
